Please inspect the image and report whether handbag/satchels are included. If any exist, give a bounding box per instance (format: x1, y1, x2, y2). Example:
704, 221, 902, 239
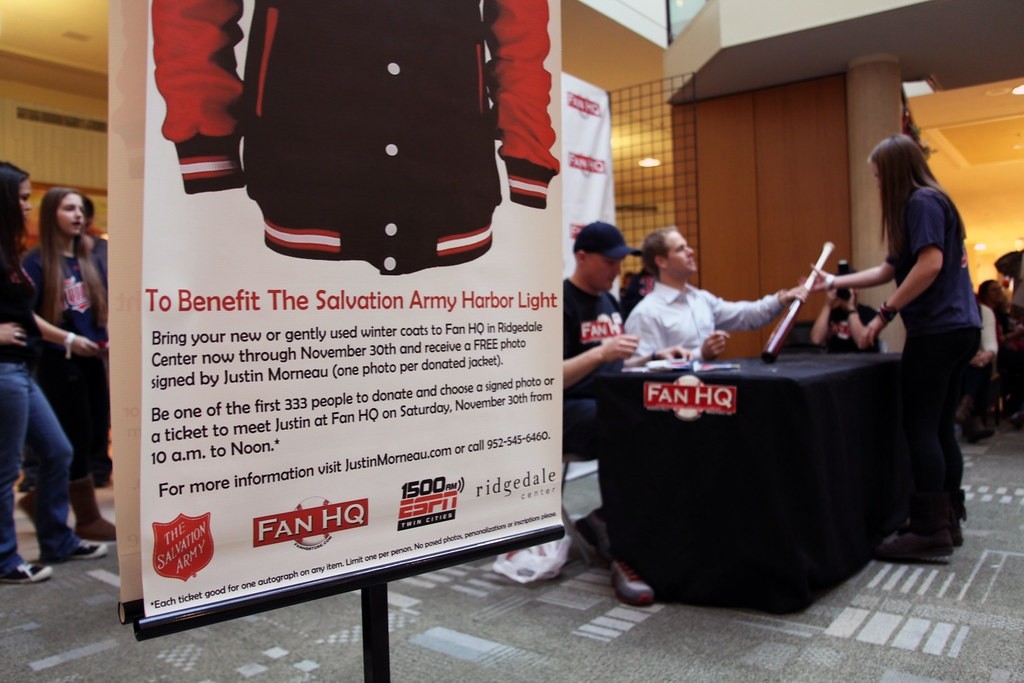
494, 528, 572, 584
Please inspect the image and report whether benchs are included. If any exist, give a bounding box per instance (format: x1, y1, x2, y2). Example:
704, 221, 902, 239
561, 450, 605, 567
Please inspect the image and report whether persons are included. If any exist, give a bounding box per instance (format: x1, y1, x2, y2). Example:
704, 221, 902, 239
563, 220, 694, 458
959, 279, 1024, 444
624, 227, 812, 362
0, 160, 116, 583
801, 135, 984, 561
620, 267, 656, 321
811, 265, 881, 354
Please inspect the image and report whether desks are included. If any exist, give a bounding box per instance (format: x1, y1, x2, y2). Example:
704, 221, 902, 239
595, 352, 908, 614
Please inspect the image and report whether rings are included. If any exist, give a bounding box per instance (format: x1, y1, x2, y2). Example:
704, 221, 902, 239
16, 332, 20, 337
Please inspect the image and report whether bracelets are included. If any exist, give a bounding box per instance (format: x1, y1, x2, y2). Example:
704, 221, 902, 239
849, 310, 857, 313
876, 302, 898, 324
64, 332, 74, 359
652, 352, 656, 360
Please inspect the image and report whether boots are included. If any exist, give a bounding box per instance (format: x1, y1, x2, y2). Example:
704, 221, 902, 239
953, 395, 995, 445
877, 490, 966, 564
67, 474, 117, 541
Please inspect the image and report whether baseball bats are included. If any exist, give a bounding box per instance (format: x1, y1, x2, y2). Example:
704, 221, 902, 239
761, 239, 837, 365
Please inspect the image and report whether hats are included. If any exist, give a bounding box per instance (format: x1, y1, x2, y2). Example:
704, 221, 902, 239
574, 222, 642, 259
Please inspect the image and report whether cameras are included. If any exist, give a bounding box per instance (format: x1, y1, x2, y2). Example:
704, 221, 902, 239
837, 259, 851, 300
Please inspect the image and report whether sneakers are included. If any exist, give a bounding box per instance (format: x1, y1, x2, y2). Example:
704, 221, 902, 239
610, 559, 656, 607
39, 540, 108, 561
0, 559, 54, 584
